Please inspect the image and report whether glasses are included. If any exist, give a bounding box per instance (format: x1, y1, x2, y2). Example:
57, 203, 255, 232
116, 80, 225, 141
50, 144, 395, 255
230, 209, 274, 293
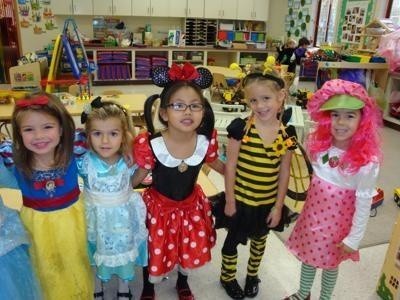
165, 101, 203, 112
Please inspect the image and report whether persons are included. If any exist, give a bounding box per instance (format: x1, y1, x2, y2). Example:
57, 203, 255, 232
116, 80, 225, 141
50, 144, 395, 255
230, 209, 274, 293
73, 97, 148, 300
290, 37, 310, 65
279, 38, 296, 65
8, 91, 95, 300
212, 64, 298, 300
131, 62, 226, 300
284, 79, 384, 300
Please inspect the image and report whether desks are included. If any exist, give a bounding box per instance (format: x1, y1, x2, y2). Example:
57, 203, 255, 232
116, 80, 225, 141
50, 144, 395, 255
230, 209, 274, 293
0, 93, 157, 137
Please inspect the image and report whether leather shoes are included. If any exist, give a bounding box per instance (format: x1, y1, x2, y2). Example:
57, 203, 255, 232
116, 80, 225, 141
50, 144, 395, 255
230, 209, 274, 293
175, 282, 194, 300
116, 288, 132, 300
244, 275, 261, 297
220, 277, 244, 299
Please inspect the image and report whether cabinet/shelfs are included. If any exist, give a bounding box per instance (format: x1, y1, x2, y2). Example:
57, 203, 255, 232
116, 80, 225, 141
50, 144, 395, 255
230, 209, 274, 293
93, 0, 132, 16
238, 0, 269, 21
170, 0, 204, 18
49, 48, 276, 86
132, 0, 169, 17
204, 0, 238, 20
181, 18, 268, 49
49, 0, 93, 15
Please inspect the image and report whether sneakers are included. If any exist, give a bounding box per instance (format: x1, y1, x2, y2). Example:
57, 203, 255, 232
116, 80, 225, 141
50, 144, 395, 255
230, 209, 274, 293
140, 287, 156, 300
93, 290, 103, 300
285, 293, 310, 300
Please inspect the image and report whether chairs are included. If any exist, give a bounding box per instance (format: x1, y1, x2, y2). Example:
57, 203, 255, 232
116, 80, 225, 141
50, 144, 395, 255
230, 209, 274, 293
205, 88, 253, 166
283, 104, 306, 144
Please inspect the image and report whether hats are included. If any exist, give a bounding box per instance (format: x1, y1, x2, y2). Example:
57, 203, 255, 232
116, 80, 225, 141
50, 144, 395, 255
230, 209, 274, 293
307, 78, 372, 123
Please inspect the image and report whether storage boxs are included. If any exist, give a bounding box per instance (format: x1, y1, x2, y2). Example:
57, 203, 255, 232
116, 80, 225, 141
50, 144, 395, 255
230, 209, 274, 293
374, 208, 400, 299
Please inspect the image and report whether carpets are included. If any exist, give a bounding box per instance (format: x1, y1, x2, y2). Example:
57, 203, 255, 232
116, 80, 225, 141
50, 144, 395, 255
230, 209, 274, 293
269, 106, 400, 261
